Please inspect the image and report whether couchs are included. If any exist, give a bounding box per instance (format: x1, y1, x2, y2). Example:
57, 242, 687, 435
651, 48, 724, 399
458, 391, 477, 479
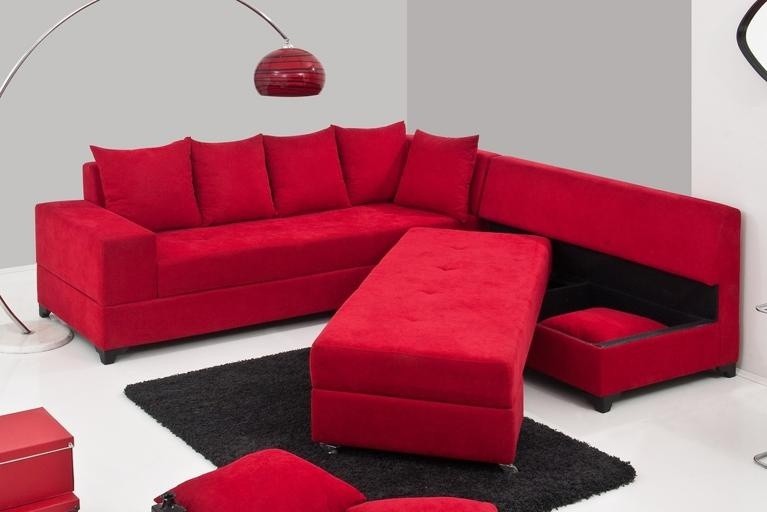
33, 135, 742, 414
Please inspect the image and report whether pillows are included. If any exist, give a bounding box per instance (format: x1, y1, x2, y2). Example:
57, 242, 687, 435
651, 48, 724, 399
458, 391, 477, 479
191, 134, 275, 226
263, 127, 352, 218
90, 137, 201, 230
394, 129, 479, 223
331, 122, 406, 206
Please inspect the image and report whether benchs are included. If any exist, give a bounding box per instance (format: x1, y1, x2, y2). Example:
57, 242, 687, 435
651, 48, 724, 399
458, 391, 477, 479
309, 226, 555, 477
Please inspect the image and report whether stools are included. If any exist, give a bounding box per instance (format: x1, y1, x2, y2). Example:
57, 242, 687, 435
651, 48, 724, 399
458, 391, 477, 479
344, 495, 498, 512
150, 449, 367, 512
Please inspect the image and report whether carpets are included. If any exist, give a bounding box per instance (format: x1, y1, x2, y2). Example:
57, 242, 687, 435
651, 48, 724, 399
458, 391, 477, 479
123, 346, 637, 512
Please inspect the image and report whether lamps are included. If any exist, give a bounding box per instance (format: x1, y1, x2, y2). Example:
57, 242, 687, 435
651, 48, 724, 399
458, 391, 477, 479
1, 1, 326, 356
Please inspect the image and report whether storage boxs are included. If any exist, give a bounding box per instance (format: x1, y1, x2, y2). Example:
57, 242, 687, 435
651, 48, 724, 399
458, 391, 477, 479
0, 409, 75, 510
